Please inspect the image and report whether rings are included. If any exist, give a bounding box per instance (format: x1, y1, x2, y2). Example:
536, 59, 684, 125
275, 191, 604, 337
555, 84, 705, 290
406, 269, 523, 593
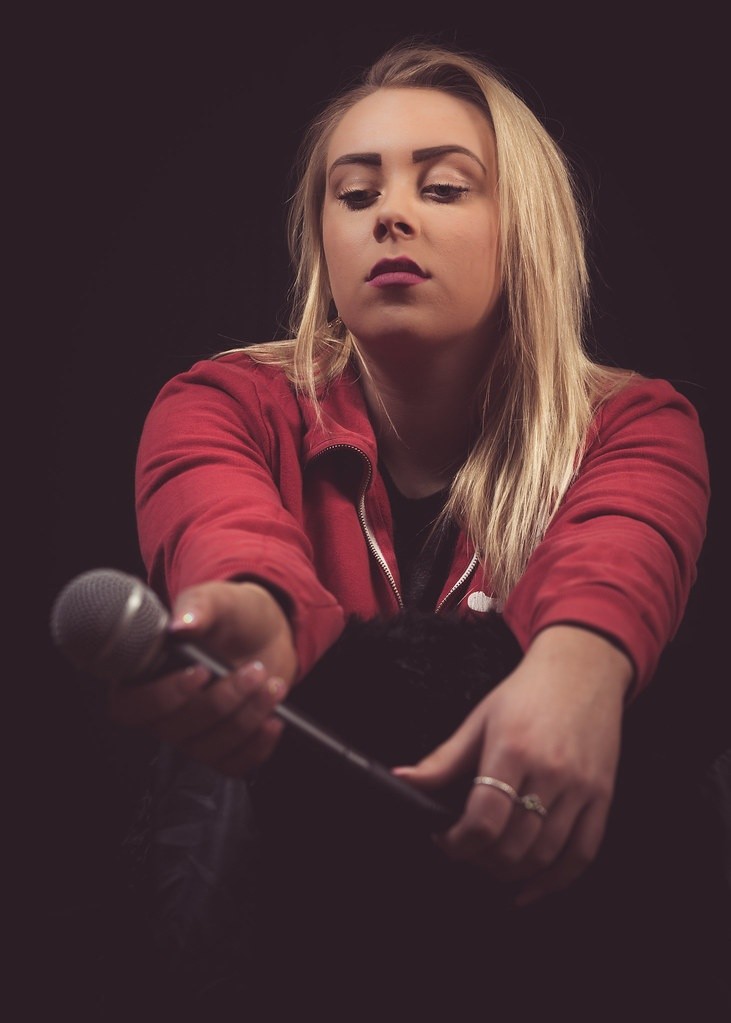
473, 776, 519, 801
518, 793, 548, 817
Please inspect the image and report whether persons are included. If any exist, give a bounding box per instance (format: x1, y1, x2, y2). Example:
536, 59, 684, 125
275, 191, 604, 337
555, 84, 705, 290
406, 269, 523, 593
105, 41, 712, 1022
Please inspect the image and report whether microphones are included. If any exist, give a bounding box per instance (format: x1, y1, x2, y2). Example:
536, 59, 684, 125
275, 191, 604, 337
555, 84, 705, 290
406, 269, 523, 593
54, 568, 460, 835
